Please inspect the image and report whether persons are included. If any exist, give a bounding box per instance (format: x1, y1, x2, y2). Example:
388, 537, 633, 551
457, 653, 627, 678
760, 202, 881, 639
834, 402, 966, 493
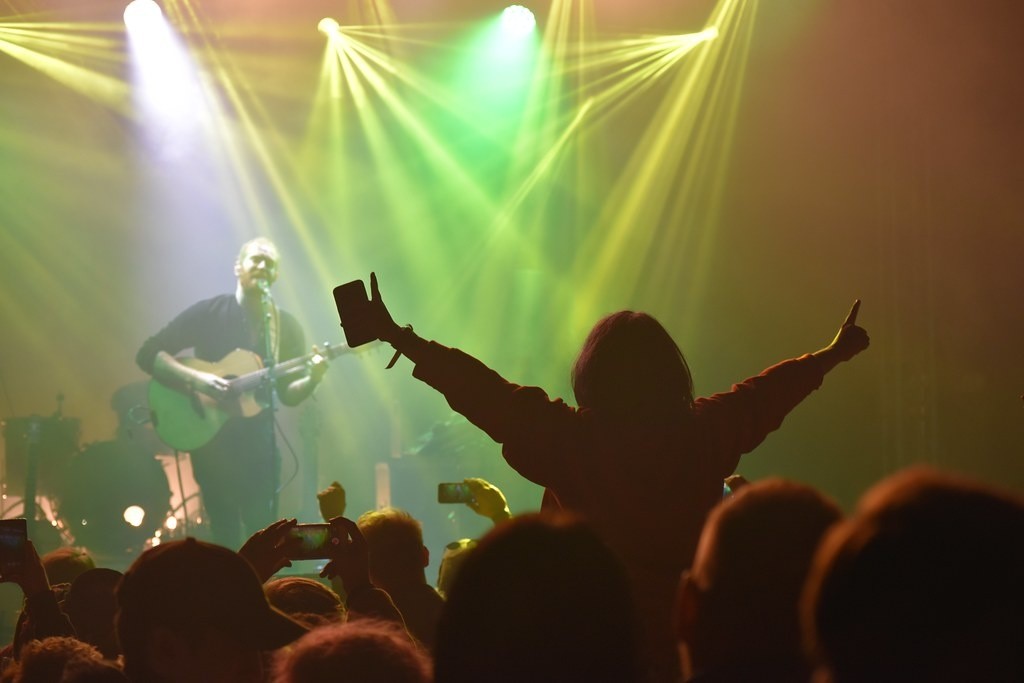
340, 272, 870, 682
0, 461, 1024, 683
137, 238, 331, 548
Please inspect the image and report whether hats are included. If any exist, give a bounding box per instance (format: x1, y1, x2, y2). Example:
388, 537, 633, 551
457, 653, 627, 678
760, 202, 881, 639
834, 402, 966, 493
112, 536, 311, 651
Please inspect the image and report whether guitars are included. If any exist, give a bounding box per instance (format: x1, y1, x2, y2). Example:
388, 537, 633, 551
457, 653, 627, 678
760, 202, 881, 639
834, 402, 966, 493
143, 334, 383, 450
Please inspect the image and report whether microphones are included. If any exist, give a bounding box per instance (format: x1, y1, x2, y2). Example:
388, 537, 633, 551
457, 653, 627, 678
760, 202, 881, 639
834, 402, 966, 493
257, 277, 273, 297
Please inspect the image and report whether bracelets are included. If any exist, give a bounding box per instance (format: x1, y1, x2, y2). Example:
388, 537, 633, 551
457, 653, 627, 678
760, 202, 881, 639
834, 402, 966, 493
384, 324, 414, 369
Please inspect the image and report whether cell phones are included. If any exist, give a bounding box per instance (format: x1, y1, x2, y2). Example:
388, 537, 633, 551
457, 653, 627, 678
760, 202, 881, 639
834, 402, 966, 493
438, 483, 476, 504
0, 517, 26, 583
283, 523, 332, 561
332, 279, 376, 347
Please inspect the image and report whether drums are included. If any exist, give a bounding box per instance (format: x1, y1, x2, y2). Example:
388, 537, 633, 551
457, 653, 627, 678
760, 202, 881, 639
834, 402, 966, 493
3, 415, 78, 497
51, 440, 174, 562
